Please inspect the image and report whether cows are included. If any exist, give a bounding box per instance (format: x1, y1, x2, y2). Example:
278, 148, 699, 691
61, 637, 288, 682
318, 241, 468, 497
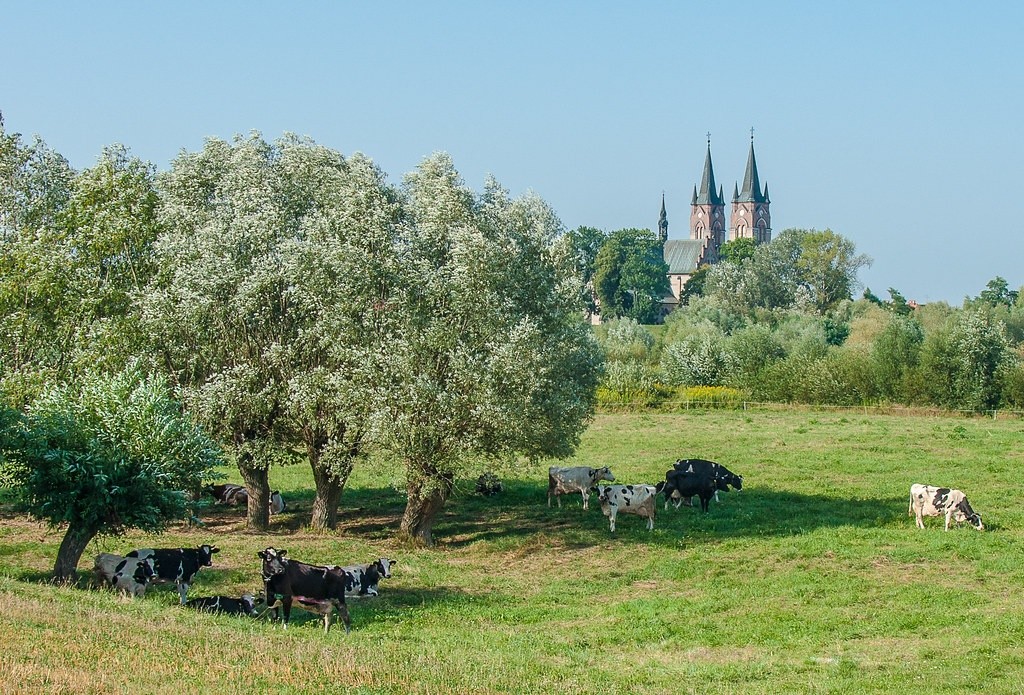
94, 544, 221, 605
546, 458, 742, 534
908, 483, 984, 532
181, 595, 265, 618
202, 482, 285, 514
256, 547, 397, 637
